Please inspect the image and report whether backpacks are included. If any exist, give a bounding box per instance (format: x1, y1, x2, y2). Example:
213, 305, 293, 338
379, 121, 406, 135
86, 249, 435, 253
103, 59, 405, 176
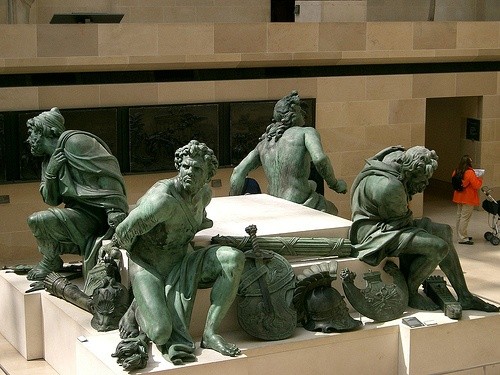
452, 168, 475, 192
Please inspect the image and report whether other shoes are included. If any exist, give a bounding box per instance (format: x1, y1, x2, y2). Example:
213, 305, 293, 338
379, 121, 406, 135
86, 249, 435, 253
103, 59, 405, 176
458, 237, 473, 245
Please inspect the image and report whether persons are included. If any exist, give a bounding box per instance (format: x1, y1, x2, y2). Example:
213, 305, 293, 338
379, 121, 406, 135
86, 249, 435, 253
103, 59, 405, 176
452, 155, 483, 245
349, 144, 500, 312
229, 90, 348, 217
25, 106, 129, 281
115, 140, 245, 357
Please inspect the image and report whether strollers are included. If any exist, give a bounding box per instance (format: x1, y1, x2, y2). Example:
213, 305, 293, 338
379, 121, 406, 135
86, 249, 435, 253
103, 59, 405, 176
480, 185, 499, 245
